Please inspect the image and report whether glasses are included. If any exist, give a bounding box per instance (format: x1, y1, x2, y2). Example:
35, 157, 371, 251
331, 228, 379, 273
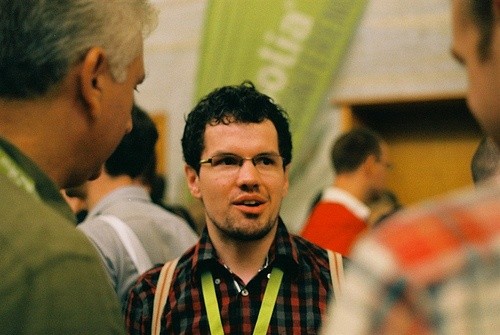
198, 153, 284, 174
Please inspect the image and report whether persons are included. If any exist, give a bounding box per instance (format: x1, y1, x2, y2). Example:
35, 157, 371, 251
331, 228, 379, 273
317, 1, 500, 335
123, 79, 355, 335
2, 0, 162, 335
65, 100, 500, 300
